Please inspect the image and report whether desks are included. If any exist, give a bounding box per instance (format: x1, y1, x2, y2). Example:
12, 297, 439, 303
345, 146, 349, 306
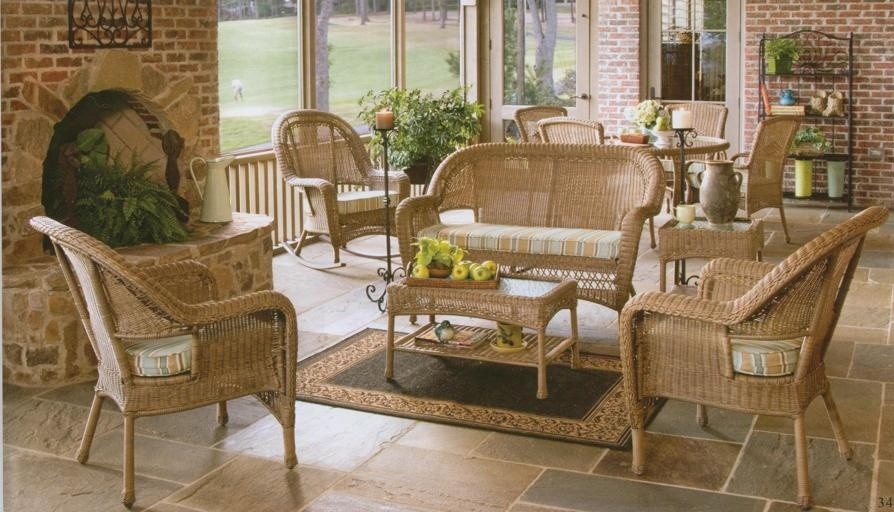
614, 135, 730, 214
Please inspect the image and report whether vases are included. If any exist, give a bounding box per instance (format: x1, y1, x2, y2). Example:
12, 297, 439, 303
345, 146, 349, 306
793, 141, 826, 158
696, 159, 744, 226
650, 132, 675, 143
794, 160, 812, 198
779, 89, 797, 105
827, 161, 846, 198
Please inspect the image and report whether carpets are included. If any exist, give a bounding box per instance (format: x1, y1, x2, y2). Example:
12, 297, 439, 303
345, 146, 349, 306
274, 327, 678, 451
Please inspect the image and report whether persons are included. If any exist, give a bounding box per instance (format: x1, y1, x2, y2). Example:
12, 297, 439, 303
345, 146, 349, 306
227, 74, 248, 107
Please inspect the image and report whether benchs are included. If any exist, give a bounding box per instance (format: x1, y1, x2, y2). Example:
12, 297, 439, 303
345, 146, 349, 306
393, 142, 664, 325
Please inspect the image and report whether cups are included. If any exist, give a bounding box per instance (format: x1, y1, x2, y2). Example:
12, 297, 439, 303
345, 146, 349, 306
670, 204, 696, 225
496, 321, 522, 348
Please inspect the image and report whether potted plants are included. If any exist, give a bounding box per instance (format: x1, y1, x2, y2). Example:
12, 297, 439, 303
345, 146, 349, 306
355, 83, 485, 184
764, 36, 799, 74
411, 235, 468, 279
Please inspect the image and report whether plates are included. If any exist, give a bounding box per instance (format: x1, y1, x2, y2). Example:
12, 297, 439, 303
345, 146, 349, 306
490, 338, 528, 353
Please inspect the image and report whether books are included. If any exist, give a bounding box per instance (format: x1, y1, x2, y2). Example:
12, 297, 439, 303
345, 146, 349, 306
760, 84, 770, 116
771, 109, 803, 112
413, 325, 491, 350
771, 113, 805, 116
770, 106, 805, 109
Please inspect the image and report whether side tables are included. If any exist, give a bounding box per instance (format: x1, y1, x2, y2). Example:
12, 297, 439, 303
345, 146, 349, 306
657, 214, 765, 290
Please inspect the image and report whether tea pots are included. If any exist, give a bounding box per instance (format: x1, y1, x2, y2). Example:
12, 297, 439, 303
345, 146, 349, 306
187, 154, 237, 223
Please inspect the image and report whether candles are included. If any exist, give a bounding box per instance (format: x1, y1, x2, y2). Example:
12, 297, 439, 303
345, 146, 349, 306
672, 108, 692, 129
374, 108, 395, 129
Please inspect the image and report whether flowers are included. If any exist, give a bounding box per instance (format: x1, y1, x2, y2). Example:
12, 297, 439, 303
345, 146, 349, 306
790, 125, 833, 150
636, 99, 674, 131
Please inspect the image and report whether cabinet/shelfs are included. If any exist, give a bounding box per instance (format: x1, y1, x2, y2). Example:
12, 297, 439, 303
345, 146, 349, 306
662, 42, 700, 100
757, 30, 852, 213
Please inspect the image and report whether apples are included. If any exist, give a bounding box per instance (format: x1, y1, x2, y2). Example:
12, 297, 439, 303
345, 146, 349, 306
451, 260, 498, 281
411, 264, 430, 280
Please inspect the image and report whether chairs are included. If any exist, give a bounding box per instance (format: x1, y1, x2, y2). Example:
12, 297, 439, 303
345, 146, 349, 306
27, 215, 298, 507
661, 100, 730, 141
537, 117, 660, 249
684, 115, 802, 244
271, 109, 411, 272
513, 105, 568, 142
618, 205, 892, 511
